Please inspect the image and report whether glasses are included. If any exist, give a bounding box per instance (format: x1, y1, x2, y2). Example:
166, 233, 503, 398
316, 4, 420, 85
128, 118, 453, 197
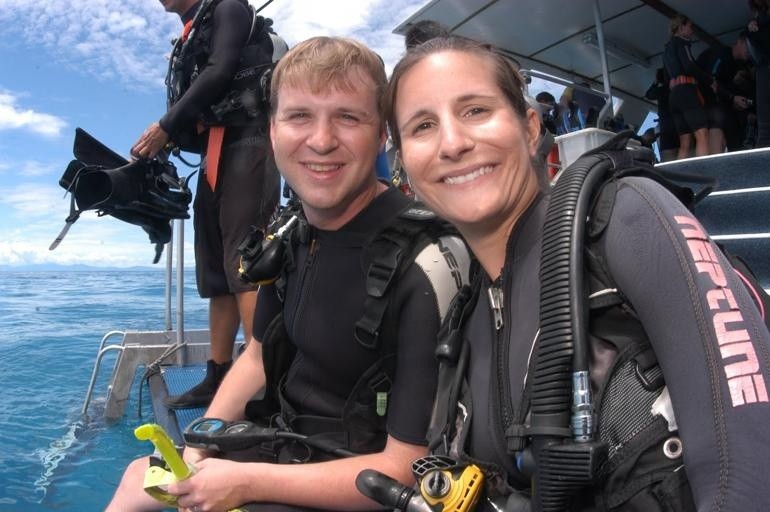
139, 465, 188, 510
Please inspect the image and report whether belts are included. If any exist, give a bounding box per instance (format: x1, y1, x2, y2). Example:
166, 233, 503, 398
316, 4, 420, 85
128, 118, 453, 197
669, 76, 700, 90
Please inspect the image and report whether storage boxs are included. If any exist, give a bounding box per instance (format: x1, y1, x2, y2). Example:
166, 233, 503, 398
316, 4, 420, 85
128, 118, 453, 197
551, 128, 619, 176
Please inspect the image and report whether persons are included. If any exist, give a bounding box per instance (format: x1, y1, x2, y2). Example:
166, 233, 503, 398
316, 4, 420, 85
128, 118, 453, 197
381, 37, 768, 511
99, 35, 471, 511
534, 0, 770, 163
120, 2, 284, 411
